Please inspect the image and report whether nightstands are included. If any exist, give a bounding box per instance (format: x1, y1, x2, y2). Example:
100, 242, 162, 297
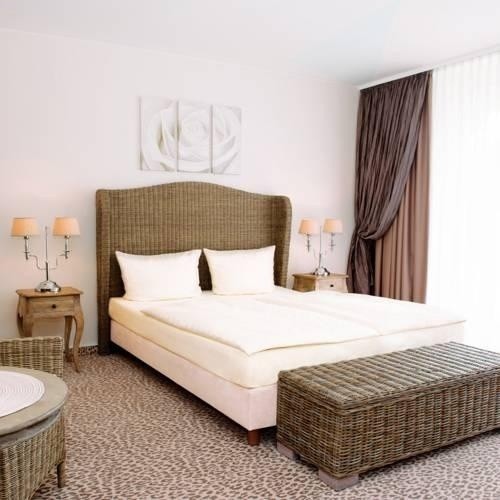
15, 287, 85, 373
292, 274, 348, 292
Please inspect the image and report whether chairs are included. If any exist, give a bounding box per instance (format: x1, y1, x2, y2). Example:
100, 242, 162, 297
0, 337, 68, 500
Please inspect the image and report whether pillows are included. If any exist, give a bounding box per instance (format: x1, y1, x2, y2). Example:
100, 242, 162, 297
115, 249, 204, 300
203, 245, 276, 294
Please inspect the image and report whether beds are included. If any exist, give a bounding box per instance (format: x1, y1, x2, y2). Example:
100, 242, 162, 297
96, 182, 466, 445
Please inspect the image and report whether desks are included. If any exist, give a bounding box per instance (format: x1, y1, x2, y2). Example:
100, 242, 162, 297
1, 365, 68, 437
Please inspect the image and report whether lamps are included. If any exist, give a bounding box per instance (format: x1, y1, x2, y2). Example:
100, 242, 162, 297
10, 218, 80, 292
298, 218, 344, 275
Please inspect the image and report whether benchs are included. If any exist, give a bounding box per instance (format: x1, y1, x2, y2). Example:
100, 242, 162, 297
276, 341, 500, 490
